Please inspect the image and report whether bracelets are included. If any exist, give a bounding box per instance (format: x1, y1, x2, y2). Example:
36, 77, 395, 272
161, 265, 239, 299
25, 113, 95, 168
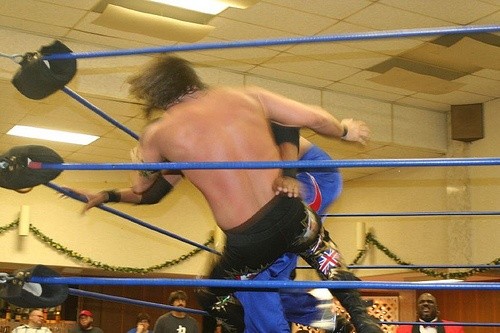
282, 168, 298, 179
342, 128, 347, 137
106, 190, 122, 203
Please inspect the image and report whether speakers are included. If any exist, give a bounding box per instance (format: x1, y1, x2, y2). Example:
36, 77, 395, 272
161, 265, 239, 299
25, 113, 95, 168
452, 102, 483, 141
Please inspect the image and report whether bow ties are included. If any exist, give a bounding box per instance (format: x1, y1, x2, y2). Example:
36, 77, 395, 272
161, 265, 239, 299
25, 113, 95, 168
424, 325, 435, 328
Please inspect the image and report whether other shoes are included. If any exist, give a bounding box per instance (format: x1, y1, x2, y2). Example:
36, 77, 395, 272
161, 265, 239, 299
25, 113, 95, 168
355, 319, 385, 333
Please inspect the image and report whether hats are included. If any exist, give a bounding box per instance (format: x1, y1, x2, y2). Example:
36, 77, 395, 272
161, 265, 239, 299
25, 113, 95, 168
79, 310, 92, 316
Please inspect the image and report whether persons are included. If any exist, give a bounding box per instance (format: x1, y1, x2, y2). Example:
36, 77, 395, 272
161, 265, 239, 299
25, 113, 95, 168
396, 293, 466, 333
56, 123, 353, 332
70, 310, 104, 333
124, 56, 386, 333
126, 312, 154, 333
154, 291, 199, 333
10, 310, 53, 333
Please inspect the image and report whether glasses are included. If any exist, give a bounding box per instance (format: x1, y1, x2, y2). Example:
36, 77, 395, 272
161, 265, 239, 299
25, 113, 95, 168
33, 315, 43, 318
